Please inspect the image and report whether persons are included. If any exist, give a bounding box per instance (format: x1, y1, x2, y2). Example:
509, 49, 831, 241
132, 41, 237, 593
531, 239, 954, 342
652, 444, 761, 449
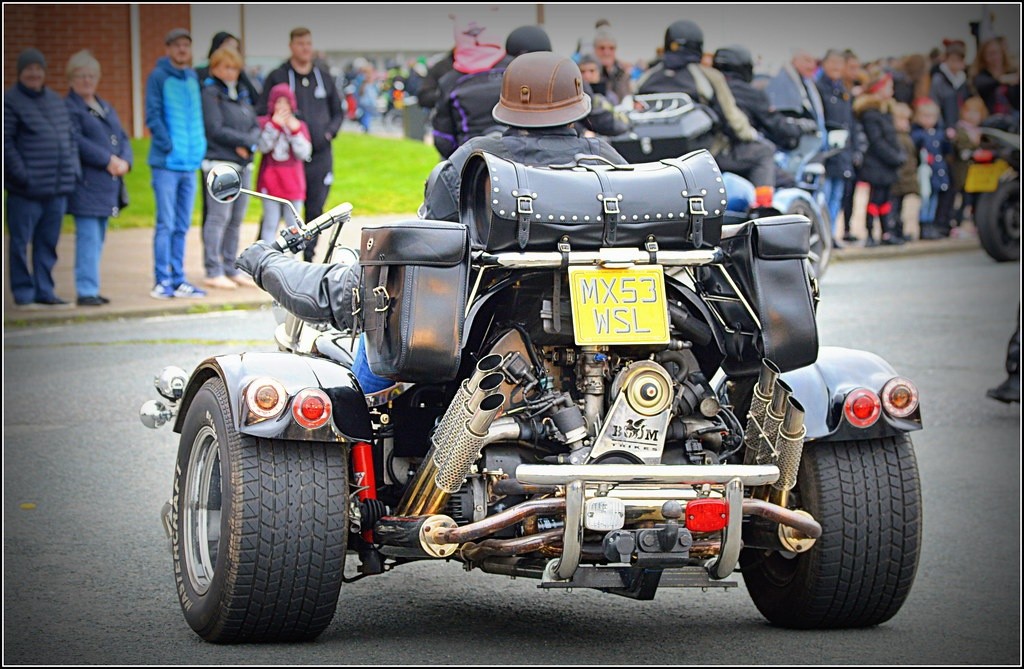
852, 69, 909, 247
425, 49, 629, 222
257, 82, 312, 245
877, 36, 1020, 238
843, 50, 861, 88
234, 239, 396, 395
631, 19, 788, 219
64, 48, 133, 306
199, 32, 267, 231
418, 47, 515, 109
145, 30, 208, 299
577, 51, 619, 110
258, 25, 343, 265
699, 44, 817, 189
201, 46, 257, 289
430, 23, 630, 159
592, 25, 630, 101
767, 45, 827, 204
3, 47, 72, 304
812, 47, 869, 250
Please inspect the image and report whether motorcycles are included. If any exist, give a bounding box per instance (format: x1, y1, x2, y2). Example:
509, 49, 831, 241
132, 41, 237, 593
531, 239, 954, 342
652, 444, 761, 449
135, 158, 924, 644
606, 66, 829, 285
963, 113, 1021, 262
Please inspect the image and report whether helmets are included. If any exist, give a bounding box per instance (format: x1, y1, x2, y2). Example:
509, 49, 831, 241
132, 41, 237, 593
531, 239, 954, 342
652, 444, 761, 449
712, 43, 754, 82
661, 20, 705, 55
453, 8, 503, 52
490, 50, 593, 127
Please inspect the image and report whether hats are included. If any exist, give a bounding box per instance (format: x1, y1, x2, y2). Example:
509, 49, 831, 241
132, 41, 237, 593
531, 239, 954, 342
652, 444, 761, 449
574, 38, 602, 67
504, 26, 552, 56
592, 18, 617, 48
961, 92, 988, 121
978, 5, 1006, 48
15, 46, 48, 75
164, 27, 193, 43
945, 39, 967, 58
63, 48, 101, 79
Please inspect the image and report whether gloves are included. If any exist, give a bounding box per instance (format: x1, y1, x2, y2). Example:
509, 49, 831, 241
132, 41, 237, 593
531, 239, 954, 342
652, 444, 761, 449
233, 238, 275, 276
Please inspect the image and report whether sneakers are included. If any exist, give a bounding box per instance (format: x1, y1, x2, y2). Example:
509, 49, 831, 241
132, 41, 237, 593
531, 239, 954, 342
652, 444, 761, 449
147, 280, 207, 300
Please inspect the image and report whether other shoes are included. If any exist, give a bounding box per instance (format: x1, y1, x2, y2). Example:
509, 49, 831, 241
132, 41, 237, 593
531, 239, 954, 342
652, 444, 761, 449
200, 274, 240, 292
76, 295, 110, 305
987, 374, 1020, 402
750, 205, 786, 220
832, 221, 963, 248
15, 295, 76, 311
229, 272, 258, 288
